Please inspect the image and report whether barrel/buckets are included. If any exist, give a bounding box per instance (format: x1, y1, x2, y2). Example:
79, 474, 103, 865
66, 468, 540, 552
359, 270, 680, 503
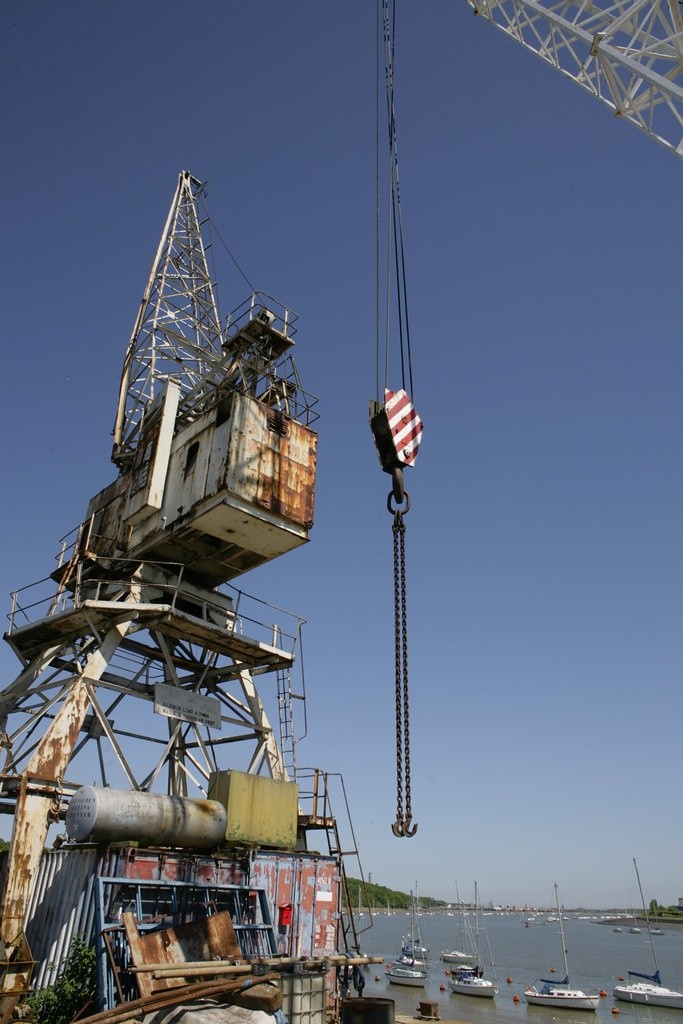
342, 997, 396, 1024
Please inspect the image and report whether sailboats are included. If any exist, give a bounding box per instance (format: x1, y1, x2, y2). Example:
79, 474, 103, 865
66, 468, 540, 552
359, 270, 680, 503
383, 880, 432, 987
338, 885, 379, 918
612, 906, 622, 933
647, 902, 665, 934
523, 883, 599, 1010
439, 880, 501, 997
612, 857, 683, 1010
625, 902, 642, 934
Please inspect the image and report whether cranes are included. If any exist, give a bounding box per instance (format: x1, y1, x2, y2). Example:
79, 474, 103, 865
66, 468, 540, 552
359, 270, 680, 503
367, 1, 683, 841
0, 164, 325, 1024
